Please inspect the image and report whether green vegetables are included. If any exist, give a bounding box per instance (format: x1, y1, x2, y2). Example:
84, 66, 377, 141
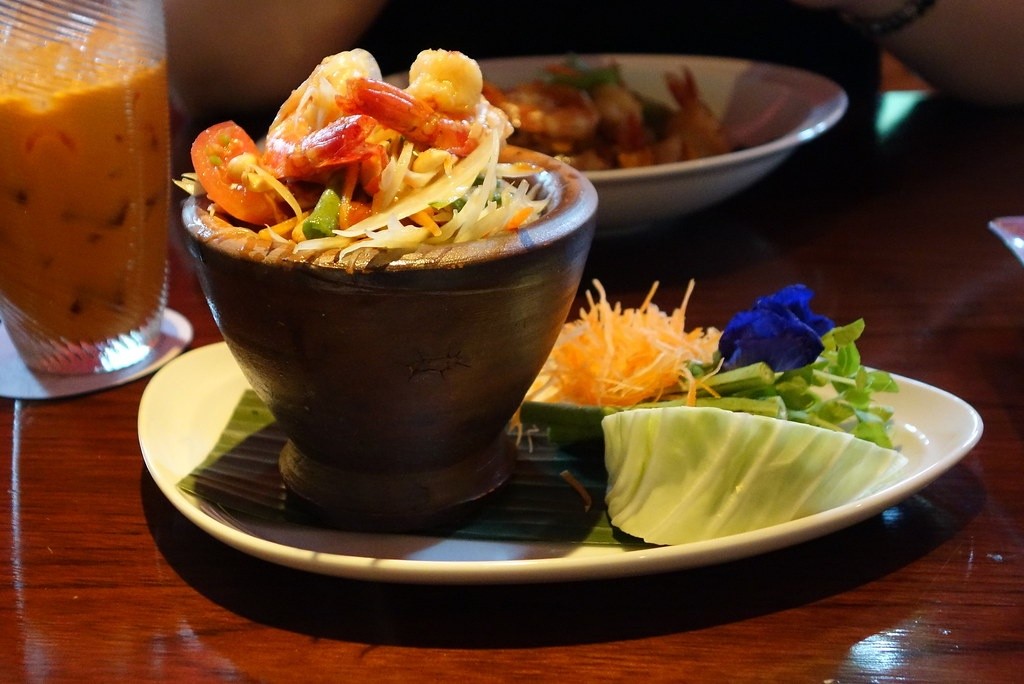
772, 318, 915, 450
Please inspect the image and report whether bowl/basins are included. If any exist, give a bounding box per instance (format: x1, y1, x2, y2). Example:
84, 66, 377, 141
177, 146, 600, 522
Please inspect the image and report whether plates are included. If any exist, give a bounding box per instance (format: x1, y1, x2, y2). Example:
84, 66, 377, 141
134, 342, 986, 583
381, 50, 850, 236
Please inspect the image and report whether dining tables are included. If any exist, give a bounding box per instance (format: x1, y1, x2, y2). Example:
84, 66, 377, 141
0, 91, 1023, 684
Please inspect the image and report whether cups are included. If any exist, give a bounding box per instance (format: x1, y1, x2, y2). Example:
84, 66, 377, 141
0, 0, 170, 377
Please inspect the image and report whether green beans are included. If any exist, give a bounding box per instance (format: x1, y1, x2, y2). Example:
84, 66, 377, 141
683, 362, 790, 421
